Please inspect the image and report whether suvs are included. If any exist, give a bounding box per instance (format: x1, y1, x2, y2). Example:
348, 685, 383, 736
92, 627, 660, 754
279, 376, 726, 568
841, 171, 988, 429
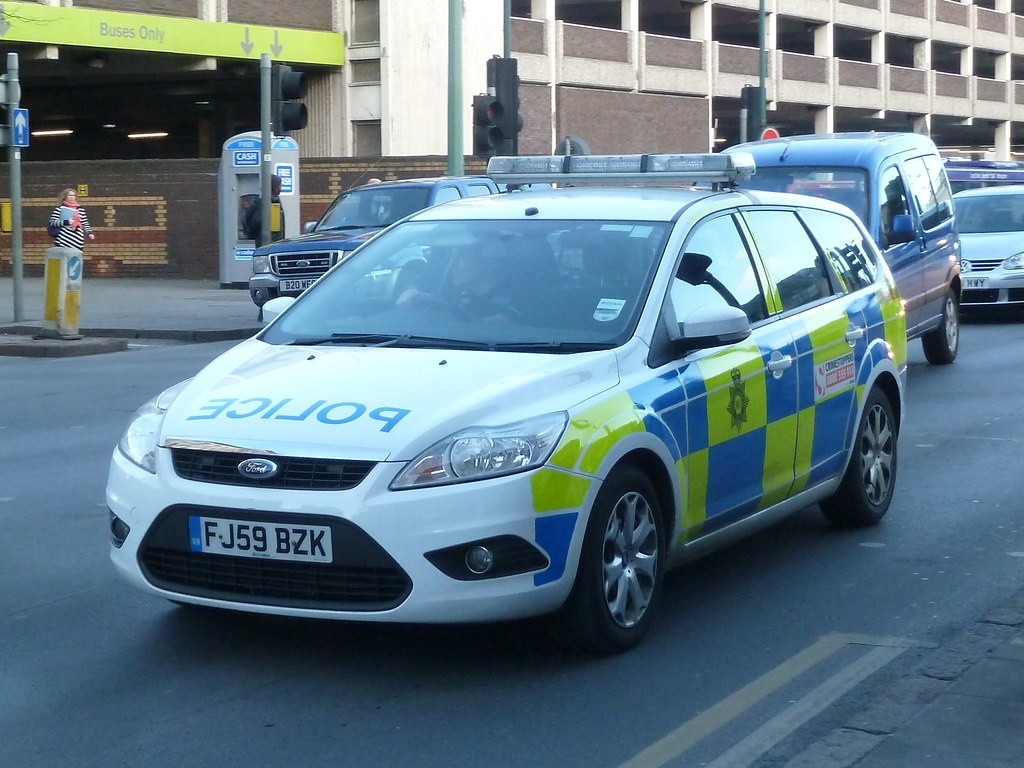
246, 173, 553, 308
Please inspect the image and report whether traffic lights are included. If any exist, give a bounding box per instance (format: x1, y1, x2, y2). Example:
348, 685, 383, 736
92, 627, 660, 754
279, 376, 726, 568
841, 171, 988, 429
269, 64, 310, 137
739, 86, 774, 143
471, 94, 506, 159
487, 58, 524, 156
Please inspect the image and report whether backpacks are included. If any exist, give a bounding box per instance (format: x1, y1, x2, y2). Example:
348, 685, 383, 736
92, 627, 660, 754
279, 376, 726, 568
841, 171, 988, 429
242, 199, 260, 240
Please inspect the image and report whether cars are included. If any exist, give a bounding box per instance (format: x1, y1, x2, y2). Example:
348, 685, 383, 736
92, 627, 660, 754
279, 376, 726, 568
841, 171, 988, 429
948, 182, 1024, 311
102, 154, 909, 657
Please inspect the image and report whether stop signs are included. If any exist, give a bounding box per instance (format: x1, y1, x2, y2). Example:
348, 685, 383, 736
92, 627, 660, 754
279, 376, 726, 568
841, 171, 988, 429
761, 128, 779, 141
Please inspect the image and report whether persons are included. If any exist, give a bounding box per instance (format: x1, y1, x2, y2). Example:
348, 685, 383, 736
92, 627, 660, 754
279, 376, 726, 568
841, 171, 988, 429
399, 245, 520, 325
49, 188, 96, 253
246, 174, 285, 249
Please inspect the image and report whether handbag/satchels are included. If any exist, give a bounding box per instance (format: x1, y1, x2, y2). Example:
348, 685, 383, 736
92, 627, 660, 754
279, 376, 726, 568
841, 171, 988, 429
47, 225, 61, 238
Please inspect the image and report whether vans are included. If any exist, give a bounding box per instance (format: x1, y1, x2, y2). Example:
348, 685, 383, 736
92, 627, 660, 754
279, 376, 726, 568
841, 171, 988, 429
689, 130, 964, 365
